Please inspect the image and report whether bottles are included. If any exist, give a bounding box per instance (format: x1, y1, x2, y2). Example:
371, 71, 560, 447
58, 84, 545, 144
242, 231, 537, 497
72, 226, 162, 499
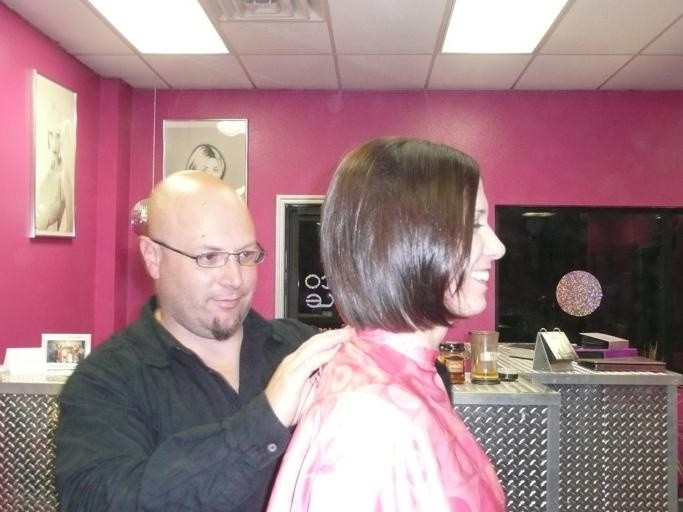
440, 342, 466, 385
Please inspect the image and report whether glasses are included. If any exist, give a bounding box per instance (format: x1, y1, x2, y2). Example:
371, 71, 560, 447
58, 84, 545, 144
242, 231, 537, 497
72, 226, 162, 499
154, 242, 268, 269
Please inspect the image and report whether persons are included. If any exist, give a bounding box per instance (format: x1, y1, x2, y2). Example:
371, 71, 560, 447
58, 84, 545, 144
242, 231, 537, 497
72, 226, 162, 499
35, 111, 73, 233
51, 168, 348, 511
47, 340, 84, 364
263, 136, 521, 512
184, 140, 245, 202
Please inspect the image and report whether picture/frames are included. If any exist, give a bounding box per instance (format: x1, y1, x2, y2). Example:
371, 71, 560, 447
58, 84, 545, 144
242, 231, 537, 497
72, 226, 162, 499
31, 69, 77, 239
162, 118, 247, 209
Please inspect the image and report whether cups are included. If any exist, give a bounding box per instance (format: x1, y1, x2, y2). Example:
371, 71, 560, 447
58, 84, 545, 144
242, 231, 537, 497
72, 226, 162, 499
470, 330, 500, 385
499, 369, 518, 381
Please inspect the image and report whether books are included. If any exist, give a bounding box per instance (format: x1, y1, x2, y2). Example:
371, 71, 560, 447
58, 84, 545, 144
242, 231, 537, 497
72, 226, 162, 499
574, 347, 638, 360
573, 356, 667, 372
577, 331, 630, 349
